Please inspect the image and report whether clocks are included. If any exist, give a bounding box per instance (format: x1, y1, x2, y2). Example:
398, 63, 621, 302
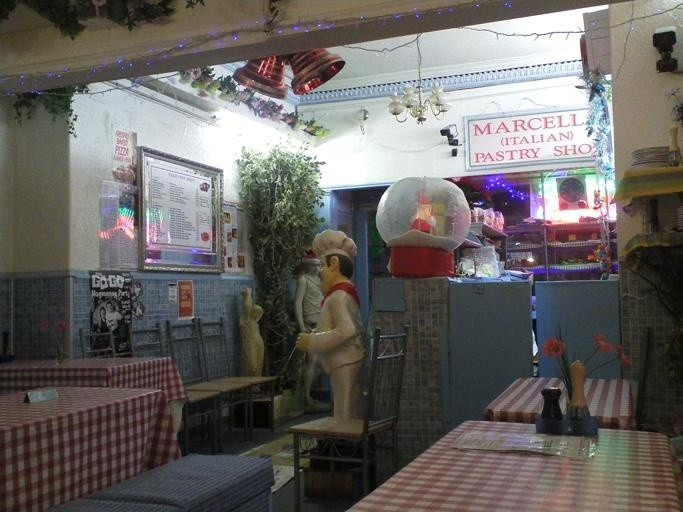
555, 174, 589, 212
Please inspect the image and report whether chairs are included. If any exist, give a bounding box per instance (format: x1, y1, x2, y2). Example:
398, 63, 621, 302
81, 319, 278, 456
286, 323, 411, 512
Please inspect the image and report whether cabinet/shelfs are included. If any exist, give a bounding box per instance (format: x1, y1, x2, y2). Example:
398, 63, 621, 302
607, 164, 682, 259
460, 217, 619, 281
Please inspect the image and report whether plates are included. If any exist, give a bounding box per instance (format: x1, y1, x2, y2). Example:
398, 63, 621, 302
630, 145, 680, 168
671, 204, 682, 231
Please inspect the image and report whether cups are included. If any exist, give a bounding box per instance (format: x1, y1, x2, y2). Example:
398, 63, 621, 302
642, 215, 652, 234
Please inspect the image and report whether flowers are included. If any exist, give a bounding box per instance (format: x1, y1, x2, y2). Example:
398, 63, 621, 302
537, 318, 632, 403
178, 65, 332, 140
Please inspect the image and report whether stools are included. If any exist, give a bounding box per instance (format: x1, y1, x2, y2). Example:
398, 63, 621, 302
43, 499, 174, 512
89, 455, 276, 512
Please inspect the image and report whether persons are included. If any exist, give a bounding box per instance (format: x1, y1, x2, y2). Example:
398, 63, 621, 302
296, 229, 365, 456
96, 301, 124, 335
238, 287, 264, 393
294, 247, 325, 407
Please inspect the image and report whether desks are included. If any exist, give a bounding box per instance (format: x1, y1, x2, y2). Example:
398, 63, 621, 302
481, 372, 636, 432
0, 386, 183, 512
0, 357, 187, 434
336, 415, 682, 512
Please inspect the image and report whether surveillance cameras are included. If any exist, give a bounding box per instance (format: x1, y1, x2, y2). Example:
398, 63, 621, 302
440, 123, 459, 146
652, 26, 678, 72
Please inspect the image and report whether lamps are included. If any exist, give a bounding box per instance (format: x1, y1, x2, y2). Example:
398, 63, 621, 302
385, 31, 453, 125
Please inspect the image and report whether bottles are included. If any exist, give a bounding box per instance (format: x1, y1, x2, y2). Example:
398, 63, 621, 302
540, 388, 562, 432
667, 125, 680, 168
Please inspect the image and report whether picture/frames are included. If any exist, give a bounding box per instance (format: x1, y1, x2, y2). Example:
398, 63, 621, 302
460, 104, 598, 172
132, 144, 227, 277
